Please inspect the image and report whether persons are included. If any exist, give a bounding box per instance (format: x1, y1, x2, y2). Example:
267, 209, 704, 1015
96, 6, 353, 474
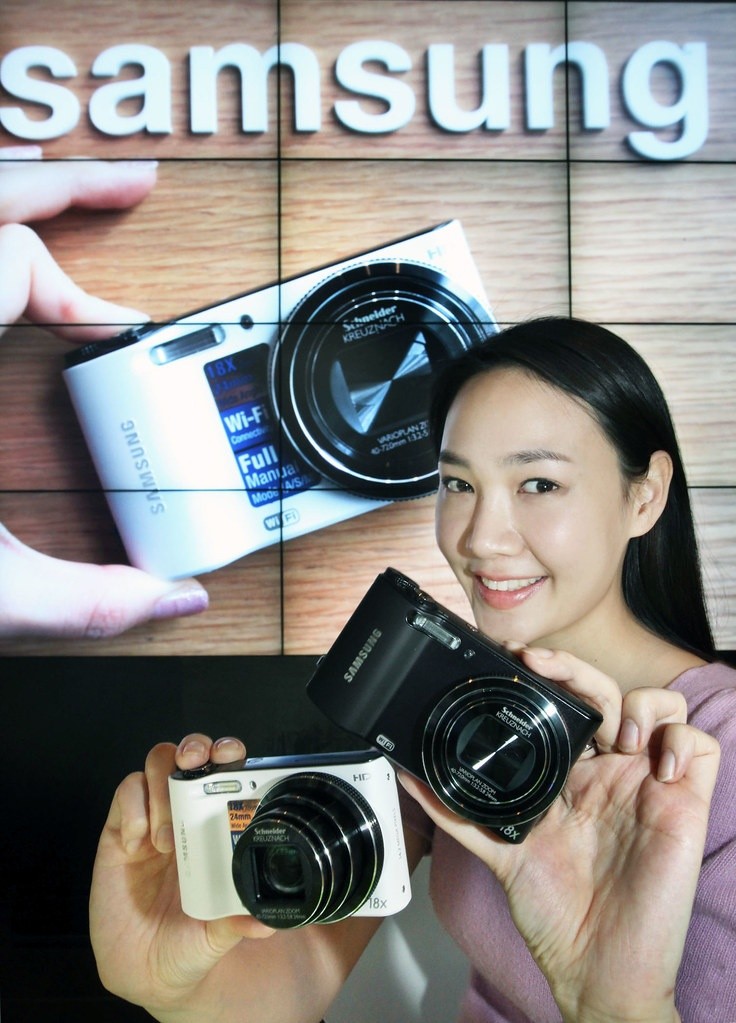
0, 143, 212, 641
88, 316, 735, 1023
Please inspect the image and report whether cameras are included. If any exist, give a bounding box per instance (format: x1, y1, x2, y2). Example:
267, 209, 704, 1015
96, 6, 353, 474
54, 219, 500, 581
306, 568, 604, 843
169, 746, 412, 929
